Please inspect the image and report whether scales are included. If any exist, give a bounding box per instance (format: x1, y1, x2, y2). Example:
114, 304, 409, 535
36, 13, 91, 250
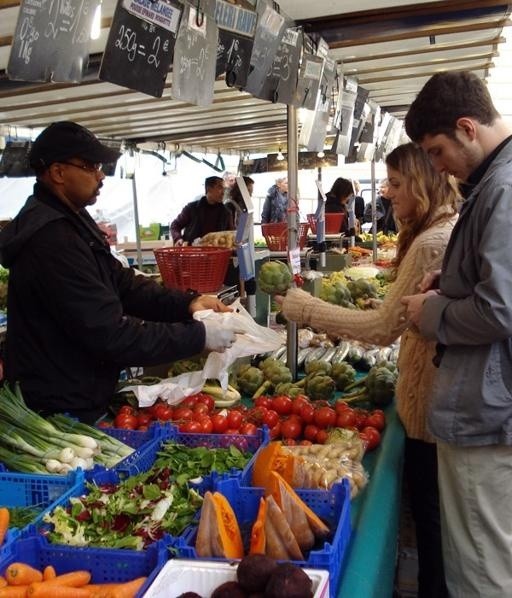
267, 179, 361, 267
199, 177, 256, 307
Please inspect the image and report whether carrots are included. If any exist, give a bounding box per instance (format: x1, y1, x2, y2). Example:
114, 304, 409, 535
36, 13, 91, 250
0, 508, 147, 598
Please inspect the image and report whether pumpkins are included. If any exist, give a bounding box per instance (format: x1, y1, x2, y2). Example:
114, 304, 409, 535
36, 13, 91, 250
196, 441, 330, 560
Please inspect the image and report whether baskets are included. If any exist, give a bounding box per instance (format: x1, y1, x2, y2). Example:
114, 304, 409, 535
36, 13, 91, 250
261, 213, 344, 251
0, 413, 352, 598
153, 246, 232, 294
0, 414, 352, 598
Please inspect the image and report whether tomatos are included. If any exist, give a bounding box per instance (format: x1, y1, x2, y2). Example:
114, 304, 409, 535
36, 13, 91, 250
99, 393, 384, 452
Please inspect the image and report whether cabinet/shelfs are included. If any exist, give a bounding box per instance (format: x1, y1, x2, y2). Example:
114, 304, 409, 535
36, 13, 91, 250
239, 371, 408, 597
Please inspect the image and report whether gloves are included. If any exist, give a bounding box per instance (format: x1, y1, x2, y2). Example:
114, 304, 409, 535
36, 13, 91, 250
189, 318, 246, 353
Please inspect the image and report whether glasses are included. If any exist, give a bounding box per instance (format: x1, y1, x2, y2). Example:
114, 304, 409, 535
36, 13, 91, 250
58, 161, 102, 172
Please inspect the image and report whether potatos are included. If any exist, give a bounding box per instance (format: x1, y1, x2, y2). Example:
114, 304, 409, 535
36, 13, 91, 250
201, 232, 235, 248
290, 444, 365, 490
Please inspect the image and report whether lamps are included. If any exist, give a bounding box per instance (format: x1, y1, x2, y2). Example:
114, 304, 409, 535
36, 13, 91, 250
277, 148, 284, 161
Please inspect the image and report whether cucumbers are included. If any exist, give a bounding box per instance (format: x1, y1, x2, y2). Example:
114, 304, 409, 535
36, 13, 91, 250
250, 342, 399, 367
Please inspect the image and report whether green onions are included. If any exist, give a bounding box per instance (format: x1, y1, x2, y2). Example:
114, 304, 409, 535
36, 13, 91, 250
0, 380, 135, 474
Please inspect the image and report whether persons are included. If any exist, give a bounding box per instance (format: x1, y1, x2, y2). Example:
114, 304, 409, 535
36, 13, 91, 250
349, 179, 365, 222
261, 172, 300, 237
272, 140, 466, 598
400, 66, 512, 598
307, 178, 363, 274
221, 175, 257, 296
5, 120, 249, 424
169, 174, 234, 251
362, 177, 400, 236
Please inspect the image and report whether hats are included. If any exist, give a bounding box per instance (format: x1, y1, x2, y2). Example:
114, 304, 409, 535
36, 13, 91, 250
30, 121, 123, 171
349, 177, 360, 197
